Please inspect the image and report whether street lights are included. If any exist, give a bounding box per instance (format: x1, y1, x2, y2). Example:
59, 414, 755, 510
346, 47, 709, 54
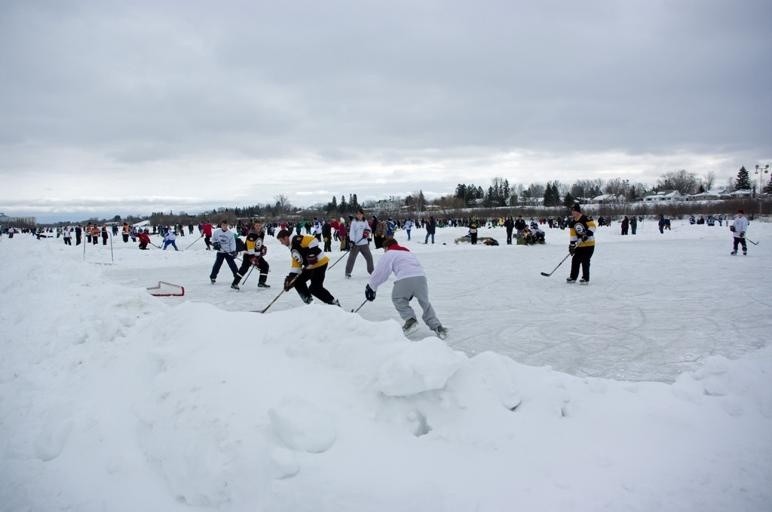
754, 163, 769, 219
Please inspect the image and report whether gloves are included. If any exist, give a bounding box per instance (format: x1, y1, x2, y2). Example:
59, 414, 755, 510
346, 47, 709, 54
260, 246, 267, 256
347, 241, 355, 250
569, 244, 576, 256
365, 284, 376, 302
582, 234, 588, 242
730, 226, 735, 232
284, 275, 295, 291
231, 252, 238, 259
740, 232, 744, 237
250, 256, 259, 267
212, 242, 221, 251
363, 231, 368, 238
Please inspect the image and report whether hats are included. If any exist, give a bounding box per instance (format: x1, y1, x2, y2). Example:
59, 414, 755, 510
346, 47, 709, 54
571, 203, 581, 213
737, 210, 744, 214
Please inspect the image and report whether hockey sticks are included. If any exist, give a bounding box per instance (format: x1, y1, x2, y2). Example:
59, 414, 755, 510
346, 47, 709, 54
743, 236, 760, 246
251, 272, 298, 314
541, 240, 583, 277
149, 243, 161, 249
352, 300, 368, 314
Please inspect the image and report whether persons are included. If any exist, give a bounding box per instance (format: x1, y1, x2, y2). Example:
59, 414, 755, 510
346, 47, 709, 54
277, 230, 340, 307
0, 210, 755, 258
344, 210, 375, 278
209, 220, 238, 283
565, 205, 596, 285
365, 239, 446, 340
230, 220, 270, 289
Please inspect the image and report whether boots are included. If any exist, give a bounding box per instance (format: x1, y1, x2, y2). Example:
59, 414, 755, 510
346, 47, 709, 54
258, 283, 270, 287
435, 326, 447, 334
231, 285, 239, 289
211, 278, 216, 282
402, 318, 416, 332
567, 278, 577, 281
345, 273, 351, 277
579, 278, 589, 282
